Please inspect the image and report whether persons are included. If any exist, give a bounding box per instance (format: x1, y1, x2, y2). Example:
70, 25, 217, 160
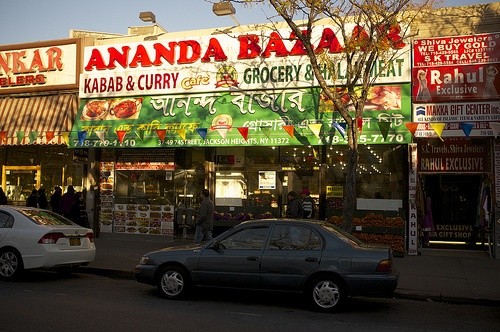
193, 189, 215, 244
37, 187, 50, 210
50, 186, 63, 215
26, 189, 39, 208
61, 186, 78, 224
286, 190, 304, 219
300, 188, 315, 219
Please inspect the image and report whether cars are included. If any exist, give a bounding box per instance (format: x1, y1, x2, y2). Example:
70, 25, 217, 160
0, 205, 96, 281
133, 219, 401, 314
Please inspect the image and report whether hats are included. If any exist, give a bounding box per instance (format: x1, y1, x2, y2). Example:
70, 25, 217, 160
301, 190, 309, 195
